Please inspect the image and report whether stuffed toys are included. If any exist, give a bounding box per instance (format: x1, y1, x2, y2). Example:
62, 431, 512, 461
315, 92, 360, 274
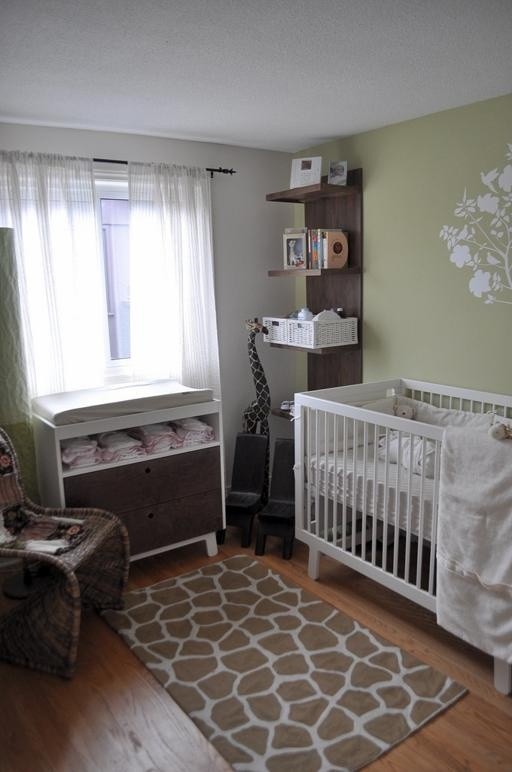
242, 319, 271, 505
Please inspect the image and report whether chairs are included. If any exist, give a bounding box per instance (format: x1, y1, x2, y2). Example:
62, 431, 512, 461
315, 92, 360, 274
217, 433, 294, 560
1, 427, 131, 679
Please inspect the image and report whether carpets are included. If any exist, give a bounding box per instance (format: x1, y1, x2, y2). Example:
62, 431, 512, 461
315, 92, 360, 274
96, 554, 471, 772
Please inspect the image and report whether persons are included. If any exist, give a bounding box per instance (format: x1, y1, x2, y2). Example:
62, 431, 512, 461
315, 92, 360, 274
330, 165, 345, 185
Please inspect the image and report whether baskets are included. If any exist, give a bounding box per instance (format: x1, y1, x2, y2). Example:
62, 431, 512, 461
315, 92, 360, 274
262, 315, 359, 350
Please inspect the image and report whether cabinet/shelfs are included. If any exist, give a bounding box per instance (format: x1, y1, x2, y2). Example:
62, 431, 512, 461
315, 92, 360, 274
30, 380, 228, 561
265, 184, 361, 420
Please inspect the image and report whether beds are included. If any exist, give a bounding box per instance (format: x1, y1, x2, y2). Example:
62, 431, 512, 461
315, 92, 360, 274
292, 380, 511, 650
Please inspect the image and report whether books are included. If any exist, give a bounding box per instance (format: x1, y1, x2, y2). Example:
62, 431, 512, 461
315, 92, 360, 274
307, 228, 349, 269
